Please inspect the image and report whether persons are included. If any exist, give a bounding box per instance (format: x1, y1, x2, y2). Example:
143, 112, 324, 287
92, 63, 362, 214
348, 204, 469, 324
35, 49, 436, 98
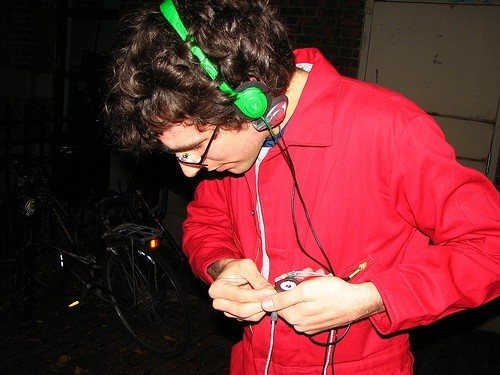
105, 0, 500, 375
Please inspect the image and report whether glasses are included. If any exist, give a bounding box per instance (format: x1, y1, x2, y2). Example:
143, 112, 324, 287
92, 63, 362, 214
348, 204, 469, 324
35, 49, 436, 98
169, 124, 219, 168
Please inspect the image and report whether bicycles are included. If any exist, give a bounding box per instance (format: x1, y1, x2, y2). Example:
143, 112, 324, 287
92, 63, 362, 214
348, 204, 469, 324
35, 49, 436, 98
0, 146, 192, 361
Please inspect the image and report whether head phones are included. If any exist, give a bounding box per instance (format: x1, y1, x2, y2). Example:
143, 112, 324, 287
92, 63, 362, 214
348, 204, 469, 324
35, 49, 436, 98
159, 0, 290, 132
